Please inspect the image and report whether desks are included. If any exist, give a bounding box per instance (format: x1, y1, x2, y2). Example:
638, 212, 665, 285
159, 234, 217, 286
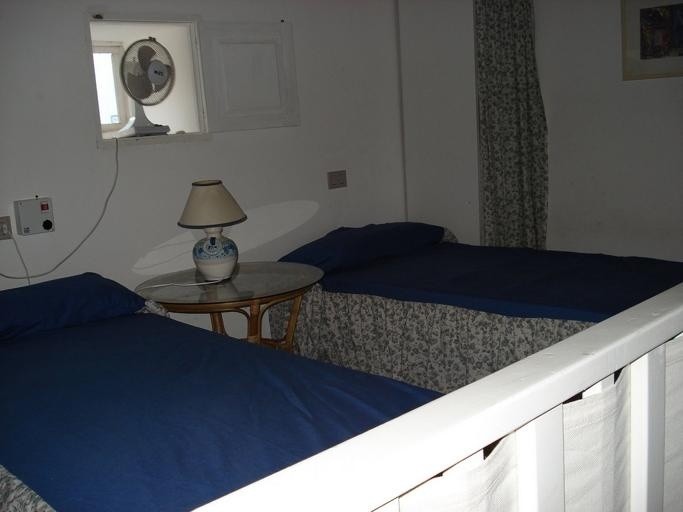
135, 260, 326, 355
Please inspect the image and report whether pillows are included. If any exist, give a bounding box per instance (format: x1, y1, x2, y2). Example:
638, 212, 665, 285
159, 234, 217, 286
1, 272, 147, 334
278, 222, 444, 279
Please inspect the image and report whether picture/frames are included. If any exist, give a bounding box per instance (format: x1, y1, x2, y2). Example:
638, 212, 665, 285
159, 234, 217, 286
621, 0, 683, 81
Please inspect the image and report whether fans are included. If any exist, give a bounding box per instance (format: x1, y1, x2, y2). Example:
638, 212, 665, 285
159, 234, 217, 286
114, 36, 175, 137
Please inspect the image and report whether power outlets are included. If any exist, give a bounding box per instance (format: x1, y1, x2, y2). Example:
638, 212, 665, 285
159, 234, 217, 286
0, 216, 12, 240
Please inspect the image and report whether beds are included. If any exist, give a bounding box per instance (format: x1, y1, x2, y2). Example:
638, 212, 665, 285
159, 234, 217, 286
0, 312, 445, 511
267, 241, 683, 393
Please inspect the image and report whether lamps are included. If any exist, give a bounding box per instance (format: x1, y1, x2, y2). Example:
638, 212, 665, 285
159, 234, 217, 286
177, 179, 247, 282
